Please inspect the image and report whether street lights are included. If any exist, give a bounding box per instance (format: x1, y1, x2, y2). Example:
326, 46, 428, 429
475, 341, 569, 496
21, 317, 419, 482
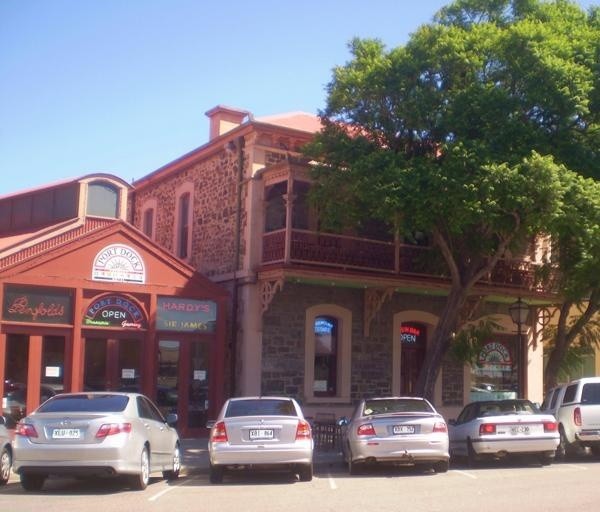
507, 295, 530, 395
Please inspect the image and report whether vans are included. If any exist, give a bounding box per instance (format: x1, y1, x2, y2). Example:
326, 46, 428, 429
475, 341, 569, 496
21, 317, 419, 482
540, 377, 600, 460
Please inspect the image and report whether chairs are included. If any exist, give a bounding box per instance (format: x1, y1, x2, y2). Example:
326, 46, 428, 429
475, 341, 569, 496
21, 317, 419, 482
314, 413, 336, 447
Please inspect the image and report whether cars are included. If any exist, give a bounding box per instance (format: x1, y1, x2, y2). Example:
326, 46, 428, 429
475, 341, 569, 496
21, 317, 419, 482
39, 382, 91, 398
471, 382, 495, 392
450, 399, 560, 469
208, 396, 315, 483
0, 391, 182, 491
338, 396, 451, 476
